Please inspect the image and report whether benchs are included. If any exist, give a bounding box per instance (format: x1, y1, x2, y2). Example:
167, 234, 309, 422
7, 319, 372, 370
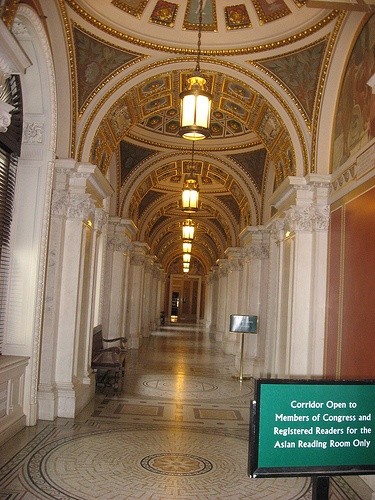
91, 323, 128, 395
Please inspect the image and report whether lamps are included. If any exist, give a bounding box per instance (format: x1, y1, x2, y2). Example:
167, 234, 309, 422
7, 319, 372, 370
179, 1, 215, 141
182, 214, 195, 273
181, 140, 200, 215
